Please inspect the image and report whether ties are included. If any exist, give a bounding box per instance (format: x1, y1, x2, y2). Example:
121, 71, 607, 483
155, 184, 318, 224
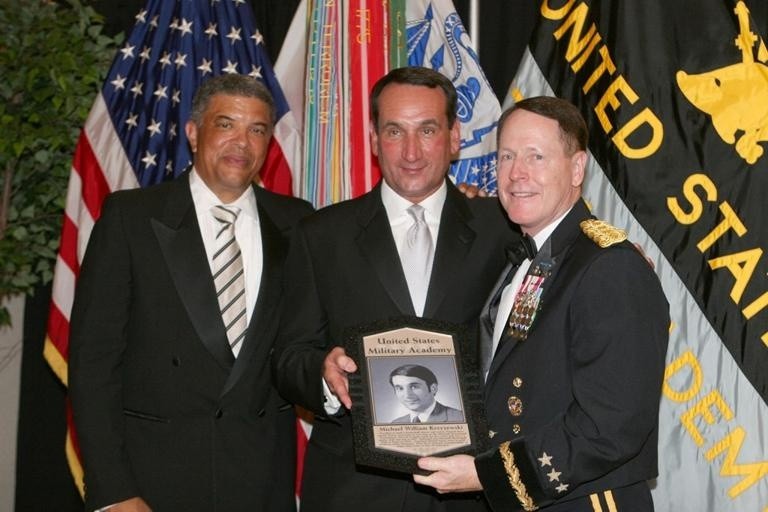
209, 205, 247, 359
400, 206, 434, 318
412, 416, 421, 422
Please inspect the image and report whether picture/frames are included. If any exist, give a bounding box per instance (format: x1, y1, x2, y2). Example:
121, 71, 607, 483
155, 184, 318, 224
344, 314, 491, 478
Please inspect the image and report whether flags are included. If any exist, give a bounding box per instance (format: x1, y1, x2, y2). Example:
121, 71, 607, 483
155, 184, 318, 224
41, 0, 316, 501
271, 2, 513, 201
478, 1, 768, 512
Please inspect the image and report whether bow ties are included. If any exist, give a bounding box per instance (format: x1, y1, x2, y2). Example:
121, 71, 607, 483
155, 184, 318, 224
505, 232, 538, 266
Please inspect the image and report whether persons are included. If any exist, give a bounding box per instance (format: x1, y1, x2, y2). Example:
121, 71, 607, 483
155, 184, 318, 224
271, 67, 520, 512
411, 97, 669, 512
382, 367, 464, 424
67, 74, 314, 512
